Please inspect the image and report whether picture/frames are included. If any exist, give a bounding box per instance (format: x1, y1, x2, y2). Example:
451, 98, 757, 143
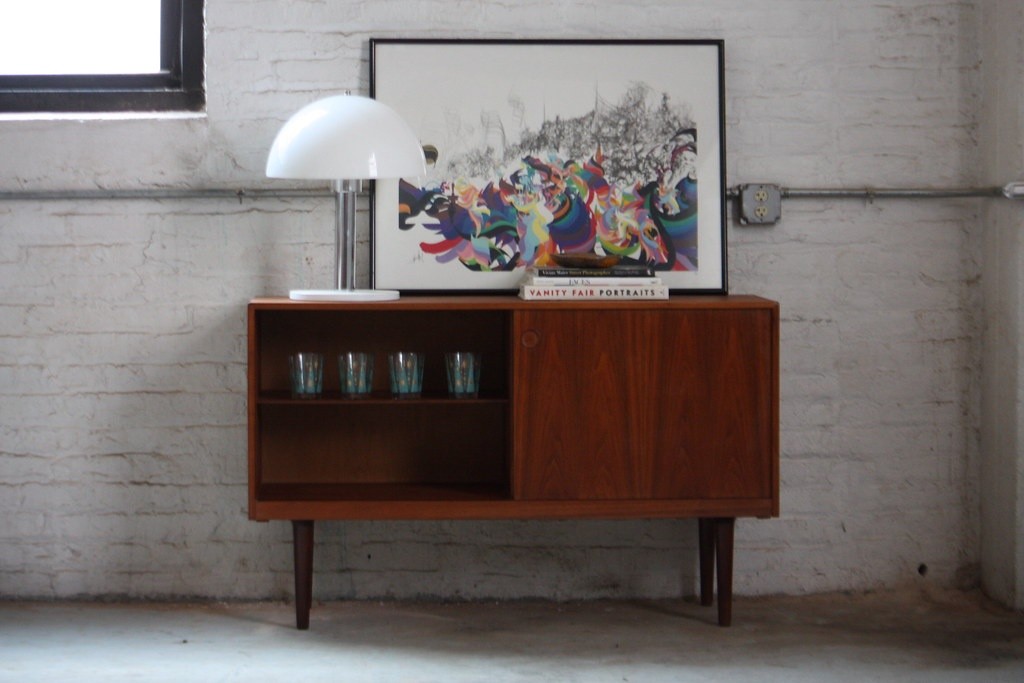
368, 39, 728, 297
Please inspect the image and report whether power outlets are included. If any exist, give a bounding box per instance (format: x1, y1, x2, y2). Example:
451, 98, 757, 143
738, 183, 780, 225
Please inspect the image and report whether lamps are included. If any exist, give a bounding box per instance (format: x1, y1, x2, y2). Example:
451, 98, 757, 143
266, 90, 428, 301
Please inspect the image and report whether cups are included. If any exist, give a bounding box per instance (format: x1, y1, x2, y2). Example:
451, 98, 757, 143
338, 350, 374, 399
388, 351, 425, 397
444, 350, 483, 397
289, 352, 325, 397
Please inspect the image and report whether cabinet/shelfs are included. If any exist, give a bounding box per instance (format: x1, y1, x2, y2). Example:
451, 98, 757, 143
246, 295, 783, 625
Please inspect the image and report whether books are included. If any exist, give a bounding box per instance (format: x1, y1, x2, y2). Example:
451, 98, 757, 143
521, 265, 669, 300
